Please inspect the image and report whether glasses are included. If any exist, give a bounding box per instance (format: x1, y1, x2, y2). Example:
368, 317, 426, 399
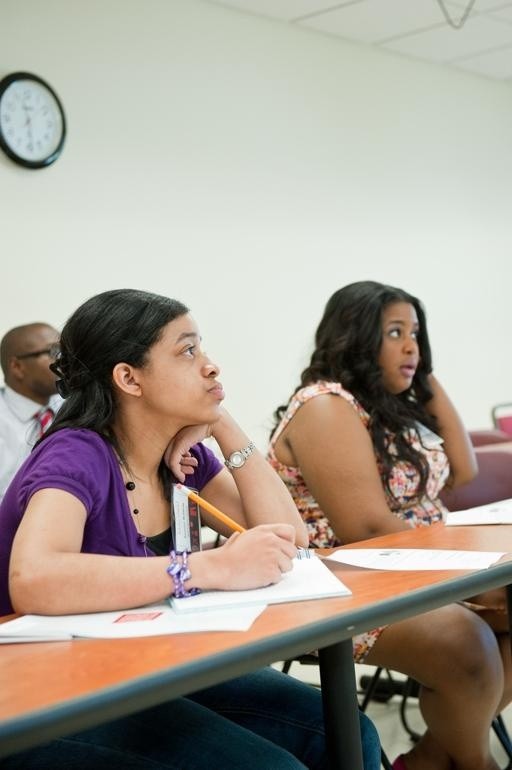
17, 346, 60, 360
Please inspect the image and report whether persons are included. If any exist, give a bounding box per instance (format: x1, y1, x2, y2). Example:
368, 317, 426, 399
1, 323, 66, 501
265, 280, 512, 769
0, 287, 380, 769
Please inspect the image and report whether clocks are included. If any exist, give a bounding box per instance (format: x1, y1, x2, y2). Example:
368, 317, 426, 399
0, 73, 66, 168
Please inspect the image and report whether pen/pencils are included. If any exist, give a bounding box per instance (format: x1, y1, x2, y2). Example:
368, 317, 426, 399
177, 482, 246, 533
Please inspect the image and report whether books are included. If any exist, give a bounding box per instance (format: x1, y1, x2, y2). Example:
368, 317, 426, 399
169, 548, 353, 614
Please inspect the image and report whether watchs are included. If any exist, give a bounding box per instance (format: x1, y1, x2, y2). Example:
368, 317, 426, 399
224, 440, 257, 471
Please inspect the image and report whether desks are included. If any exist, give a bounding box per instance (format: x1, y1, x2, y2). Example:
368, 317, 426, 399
0, 496, 512, 770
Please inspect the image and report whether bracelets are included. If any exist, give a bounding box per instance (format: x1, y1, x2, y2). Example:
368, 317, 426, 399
168, 551, 199, 600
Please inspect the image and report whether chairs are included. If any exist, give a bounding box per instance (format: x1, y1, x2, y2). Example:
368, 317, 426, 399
283, 404, 512, 770
